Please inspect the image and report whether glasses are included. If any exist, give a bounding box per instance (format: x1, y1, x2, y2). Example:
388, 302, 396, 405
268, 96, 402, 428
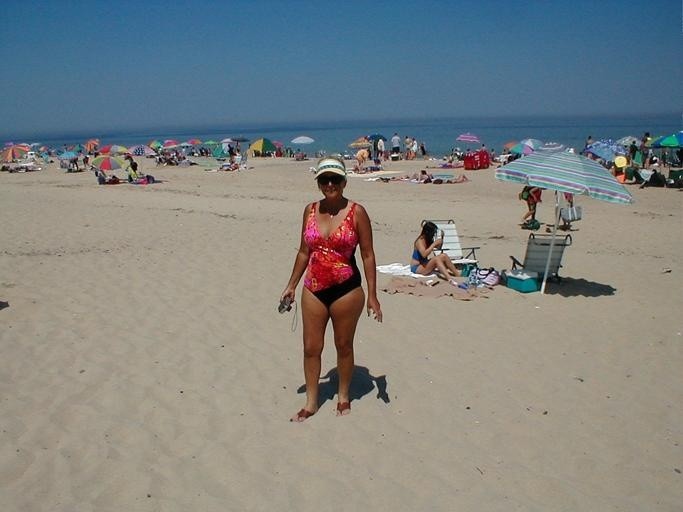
318, 175, 344, 184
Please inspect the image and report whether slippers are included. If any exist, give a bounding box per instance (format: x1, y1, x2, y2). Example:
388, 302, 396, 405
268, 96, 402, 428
337, 400, 351, 415
290, 408, 314, 422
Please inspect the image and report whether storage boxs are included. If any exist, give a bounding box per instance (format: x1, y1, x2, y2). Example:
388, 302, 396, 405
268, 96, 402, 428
505, 271, 539, 293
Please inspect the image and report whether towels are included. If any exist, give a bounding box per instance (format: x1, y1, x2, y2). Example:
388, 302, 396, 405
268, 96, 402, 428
375, 263, 437, 278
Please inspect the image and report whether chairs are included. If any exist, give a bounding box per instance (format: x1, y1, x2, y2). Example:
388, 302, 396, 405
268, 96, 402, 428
665, 169, 683, 188
351, 160, 366, 174
391, 156, 400, 161
510, 233, 572, 284
421, 219, 480, 270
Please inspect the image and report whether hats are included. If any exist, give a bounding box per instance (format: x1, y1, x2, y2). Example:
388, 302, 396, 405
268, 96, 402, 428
314, 159, 346, 179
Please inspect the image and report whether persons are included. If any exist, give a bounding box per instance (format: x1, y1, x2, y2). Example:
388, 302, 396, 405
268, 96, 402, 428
1, 135, 315, 184
347, 130, 683, 227
411, 221, 461, 287
280, 154, 382, 422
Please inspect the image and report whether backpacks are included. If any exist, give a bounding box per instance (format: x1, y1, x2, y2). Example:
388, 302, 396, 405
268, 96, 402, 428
519, 186, 535, 201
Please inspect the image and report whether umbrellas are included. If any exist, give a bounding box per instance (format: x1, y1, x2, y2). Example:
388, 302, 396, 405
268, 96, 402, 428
489, 147, 632, 291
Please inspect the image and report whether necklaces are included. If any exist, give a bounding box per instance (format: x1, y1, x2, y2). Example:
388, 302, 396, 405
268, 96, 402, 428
327, 212, 336, 220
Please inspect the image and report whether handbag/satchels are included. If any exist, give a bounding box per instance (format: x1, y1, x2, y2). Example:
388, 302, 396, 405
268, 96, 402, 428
523, 219, 540, 230
470, 268, 500, 286
561, 207, 581, 221
